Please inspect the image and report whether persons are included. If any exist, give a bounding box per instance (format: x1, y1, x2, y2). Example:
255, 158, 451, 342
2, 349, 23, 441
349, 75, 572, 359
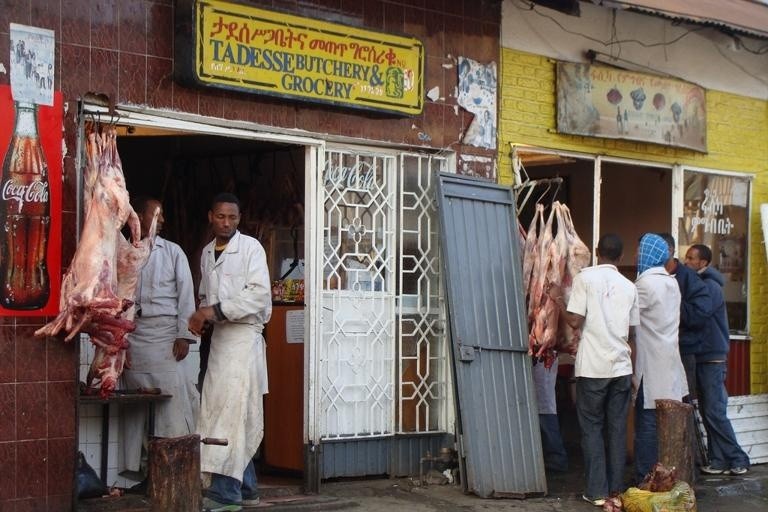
546, 233, 639, 506
535, 356, 566, 492
657, 234, 715, 403
631, 233, 689, 488
120, 198, 196, 479
684, 243, 750, 476
189, 194, 271, 512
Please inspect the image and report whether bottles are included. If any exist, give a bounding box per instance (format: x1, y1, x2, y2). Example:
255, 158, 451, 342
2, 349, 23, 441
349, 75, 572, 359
0, 99, 50, 310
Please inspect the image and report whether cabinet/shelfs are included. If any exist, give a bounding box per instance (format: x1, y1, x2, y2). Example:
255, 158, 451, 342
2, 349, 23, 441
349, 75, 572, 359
264, 291, 429, 477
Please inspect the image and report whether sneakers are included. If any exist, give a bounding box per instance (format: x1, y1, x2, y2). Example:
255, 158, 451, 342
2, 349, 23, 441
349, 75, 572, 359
244, 495, 261, 507
728, 466, 749, 475
584, 494, 606, 505
702, 464, 730, 475
201, 496, 242, 512
